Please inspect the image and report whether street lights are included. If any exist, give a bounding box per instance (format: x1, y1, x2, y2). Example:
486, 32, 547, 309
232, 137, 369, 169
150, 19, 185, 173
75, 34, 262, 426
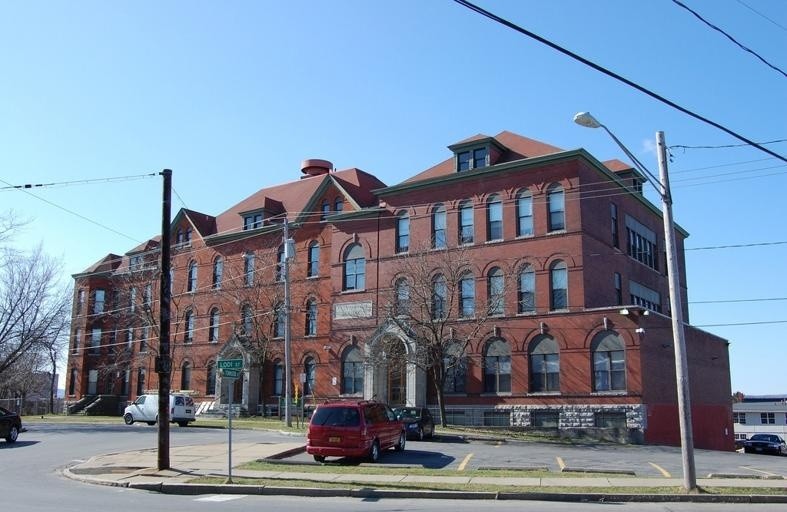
571, 108, 701, 493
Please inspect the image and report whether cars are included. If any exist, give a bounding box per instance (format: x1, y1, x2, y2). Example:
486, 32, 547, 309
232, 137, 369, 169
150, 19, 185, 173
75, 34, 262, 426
743, 432, 787, 456
393, 406, 436, 441
0, 406, 23, 443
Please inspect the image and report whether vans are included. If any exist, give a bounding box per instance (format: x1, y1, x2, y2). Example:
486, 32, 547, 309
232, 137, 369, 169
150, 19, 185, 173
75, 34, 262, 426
122, 392, 197, 427
304, 397, 406, 463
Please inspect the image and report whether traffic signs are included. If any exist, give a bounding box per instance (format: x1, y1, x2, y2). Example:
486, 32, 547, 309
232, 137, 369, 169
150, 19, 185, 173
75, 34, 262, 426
217, 360, 245, 369
220, 368, 242, 380
